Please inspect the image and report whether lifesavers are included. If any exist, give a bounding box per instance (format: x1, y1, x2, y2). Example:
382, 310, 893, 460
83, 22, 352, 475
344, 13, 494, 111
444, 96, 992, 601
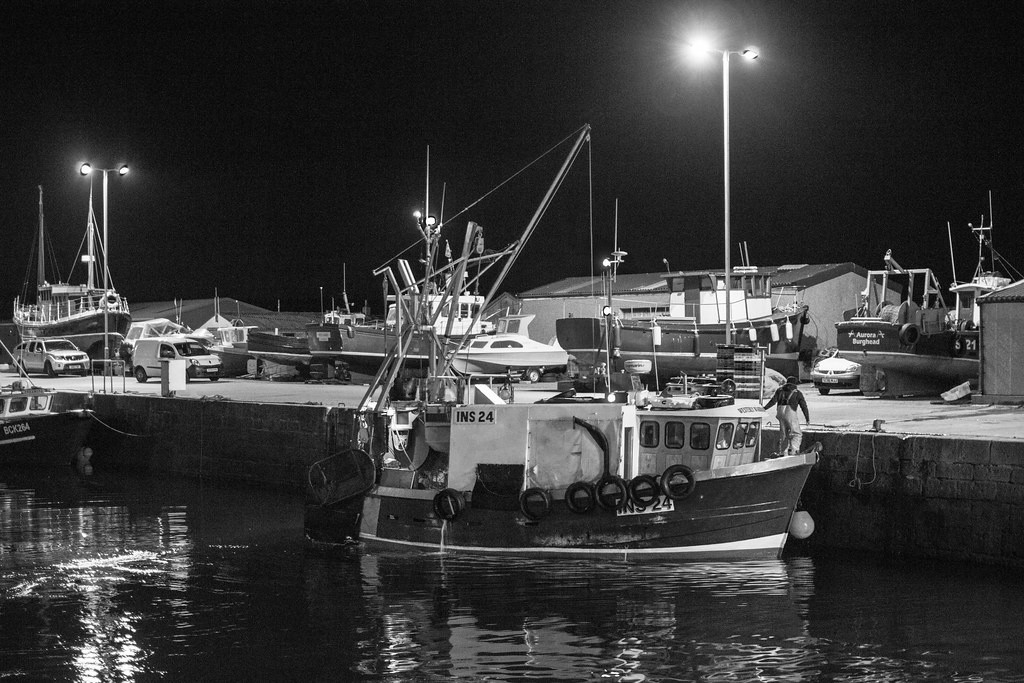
594, 475, 630, 510
660, 464, 696, 500
434, 488, 465, 521
519, 487, 553, 521
628, 475, 660, 506
899, 323, 920, 346
565, 482, 596, 513
949, 335, 967, 357
104, 291, 119, 308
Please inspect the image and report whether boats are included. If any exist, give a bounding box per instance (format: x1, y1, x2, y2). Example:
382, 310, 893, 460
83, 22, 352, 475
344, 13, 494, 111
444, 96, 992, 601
356, 123, 824, 564
811, 349, 864, 396
120, 143, 570, 385
12, 169, 131, 375
833, 191, 1024, 400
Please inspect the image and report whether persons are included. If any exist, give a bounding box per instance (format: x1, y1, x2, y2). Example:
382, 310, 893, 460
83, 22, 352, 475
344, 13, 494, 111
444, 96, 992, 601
763, 376, 810, 456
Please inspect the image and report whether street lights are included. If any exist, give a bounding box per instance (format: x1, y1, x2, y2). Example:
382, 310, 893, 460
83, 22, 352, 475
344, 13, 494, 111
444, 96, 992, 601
412, 210, 437, 307
691, 40, 762, 344
80, 165, 130, 377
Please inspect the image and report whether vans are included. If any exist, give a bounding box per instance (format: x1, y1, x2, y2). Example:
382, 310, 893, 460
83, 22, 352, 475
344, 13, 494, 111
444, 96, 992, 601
132, 336, 224, 384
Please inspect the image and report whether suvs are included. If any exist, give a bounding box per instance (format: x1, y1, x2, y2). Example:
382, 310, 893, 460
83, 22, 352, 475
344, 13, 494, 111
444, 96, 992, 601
12, 337, 91, 379
